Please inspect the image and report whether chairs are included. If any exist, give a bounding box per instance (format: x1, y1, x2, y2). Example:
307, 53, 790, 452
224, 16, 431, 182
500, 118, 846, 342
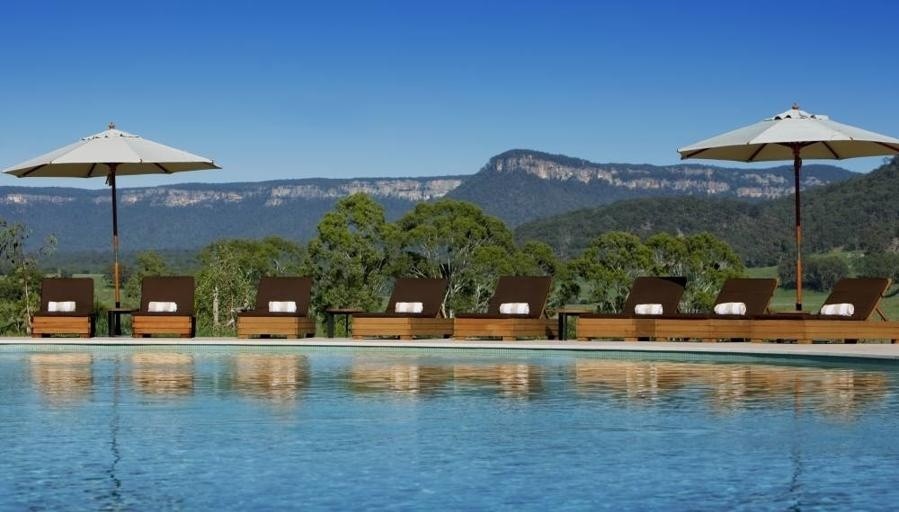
235, 278, 316, 341
352, 278, 456, 341
130, 277, 198, 339
576, 278, 684, 343
751, 276, 898, 344
32, 277, 96, 339
450, 277, 559, 342
652, 278, 777, 342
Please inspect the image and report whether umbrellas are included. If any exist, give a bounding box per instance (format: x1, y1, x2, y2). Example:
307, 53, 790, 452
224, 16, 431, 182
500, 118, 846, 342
676, 99, 899, 259
2, 122, 226, 254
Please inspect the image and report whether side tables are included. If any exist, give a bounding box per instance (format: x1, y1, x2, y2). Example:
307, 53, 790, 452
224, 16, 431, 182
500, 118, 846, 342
105, 306, 139, 338
325, 306, 363, 339
556, 304, 593, 342
